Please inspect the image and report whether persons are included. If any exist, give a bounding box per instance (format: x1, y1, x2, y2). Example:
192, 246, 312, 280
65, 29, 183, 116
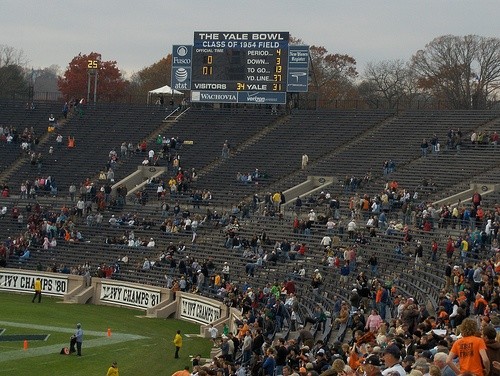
103, 134, 186, 167
389, 160, 395, 173
302, 152, 308, 170
419, 134, 440, 156
61, 96, 94, 117
222, 140, 231, 157
49, 146, 54, 156
48, 114, 56, 126
0, 123, 43, 167
67, 136, 76, 150
383, 160, 387, 175
155, 93, 188, 112
56, 134, 63, 146
267, 96, 299, 114
464, 129, 500, 145
446, 129, 462, 143
0, 168, 500, 376
47, 126, 56, 140
25, 102, 39, 110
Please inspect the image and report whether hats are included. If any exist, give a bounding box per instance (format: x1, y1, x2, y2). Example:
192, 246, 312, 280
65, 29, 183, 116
302, 345, 309, 349
77, 323, 83, 326
418, 350, 433, 359
317, 348, 325, 354
300, 367, 306, 372
306, 362, 313, 368
379, 344, 400, 354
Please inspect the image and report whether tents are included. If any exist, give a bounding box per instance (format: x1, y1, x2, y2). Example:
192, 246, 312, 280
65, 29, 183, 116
146, 85, 184, 109
